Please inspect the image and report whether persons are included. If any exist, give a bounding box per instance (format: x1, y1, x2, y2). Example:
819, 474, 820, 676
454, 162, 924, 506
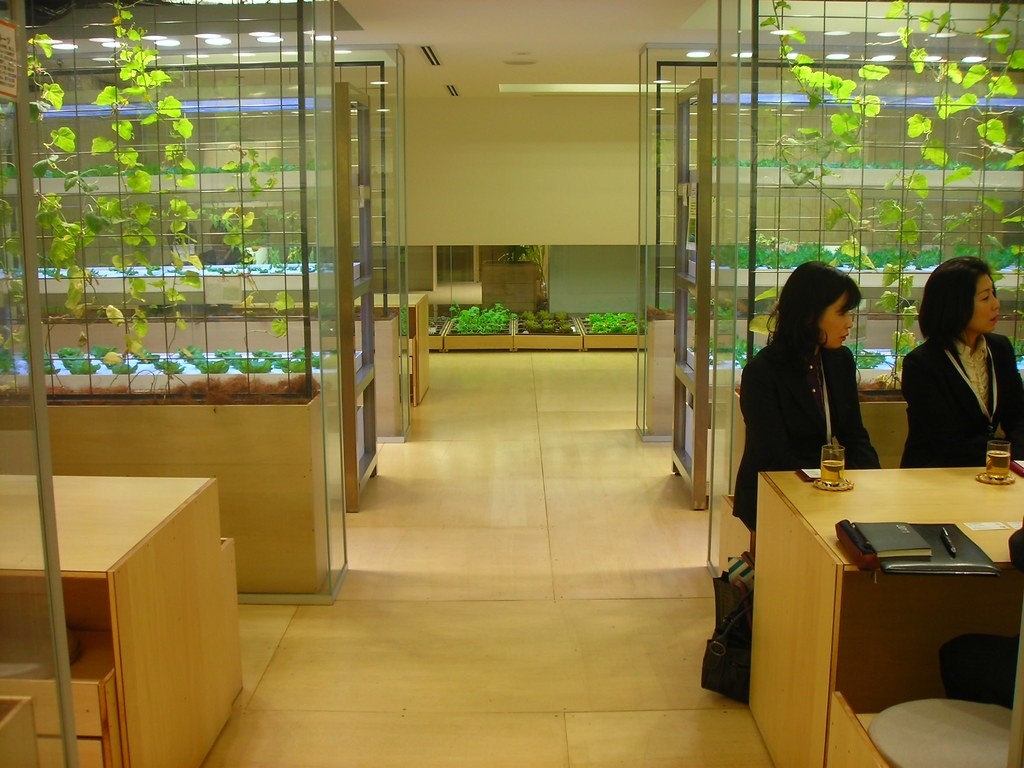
900, 256, 1024, 468
939, 515, 1024, 711
733, 261, 880, 530
160, 220, 253, 266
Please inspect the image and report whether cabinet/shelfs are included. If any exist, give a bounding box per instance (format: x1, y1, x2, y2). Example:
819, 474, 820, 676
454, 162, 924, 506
672, 78, 1024, 511
0, 81, 377, 513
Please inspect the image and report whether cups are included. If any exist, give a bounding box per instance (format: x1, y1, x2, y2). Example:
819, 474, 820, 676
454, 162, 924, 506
821, 444, 846, 487
986, 441, 1012, 480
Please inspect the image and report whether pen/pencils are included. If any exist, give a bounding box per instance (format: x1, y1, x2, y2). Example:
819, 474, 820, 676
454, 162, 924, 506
941, 525, 957, 555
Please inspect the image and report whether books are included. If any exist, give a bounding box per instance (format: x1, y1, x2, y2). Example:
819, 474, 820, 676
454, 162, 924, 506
1010, 461, 1024, 476
854, 522, 1000, 577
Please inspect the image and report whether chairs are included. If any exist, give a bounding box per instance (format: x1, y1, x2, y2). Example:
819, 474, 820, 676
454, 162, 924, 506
867, 516, 1024, 768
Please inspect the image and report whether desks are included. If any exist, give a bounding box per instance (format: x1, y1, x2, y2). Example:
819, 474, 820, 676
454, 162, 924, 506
0, 474, 243, 768
748, 467, 1024, 768
236, 294, 429, 408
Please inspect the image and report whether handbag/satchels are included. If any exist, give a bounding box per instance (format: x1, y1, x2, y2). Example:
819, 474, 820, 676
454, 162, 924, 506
701, 551, 756, 705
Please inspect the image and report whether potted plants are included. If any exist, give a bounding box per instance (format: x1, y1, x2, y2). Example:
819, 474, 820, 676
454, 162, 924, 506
429, 299, 646, 352
482, 245, 546, 313
